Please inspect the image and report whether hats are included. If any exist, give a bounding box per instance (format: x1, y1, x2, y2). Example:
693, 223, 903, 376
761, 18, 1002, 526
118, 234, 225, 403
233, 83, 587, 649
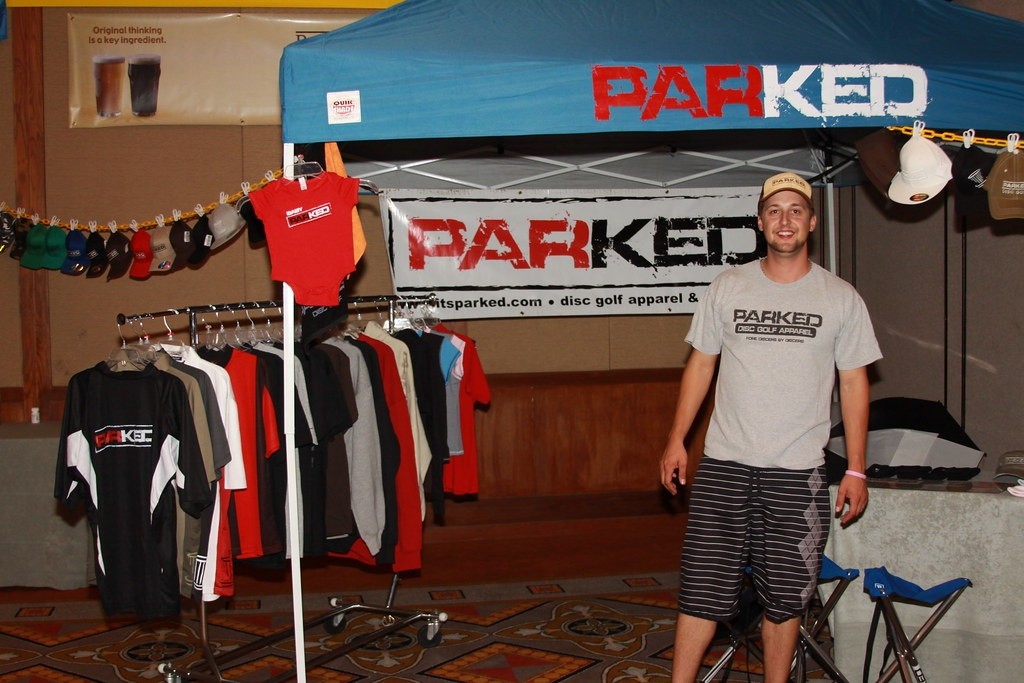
0, 196, 266, 281
983, 149, 1024, 220
758, 172, 814, 208
951, 145, 998, 212
854, 128, 900, 198
888, 135, 953, 205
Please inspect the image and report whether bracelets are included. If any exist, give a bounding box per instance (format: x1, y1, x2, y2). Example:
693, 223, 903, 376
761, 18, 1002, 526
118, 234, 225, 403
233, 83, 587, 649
845, 470, 866, 479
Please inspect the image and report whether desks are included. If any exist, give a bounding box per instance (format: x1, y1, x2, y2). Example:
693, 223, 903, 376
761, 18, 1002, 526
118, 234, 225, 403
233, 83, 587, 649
821, 469, 1024, 683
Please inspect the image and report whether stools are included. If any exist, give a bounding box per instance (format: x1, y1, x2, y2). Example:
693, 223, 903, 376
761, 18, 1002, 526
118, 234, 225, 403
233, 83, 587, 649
863, 565, 973, 683
702, 551, 859, 683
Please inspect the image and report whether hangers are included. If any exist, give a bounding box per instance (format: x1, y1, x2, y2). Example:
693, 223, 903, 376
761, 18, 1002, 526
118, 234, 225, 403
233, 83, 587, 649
273, 156, 323, 191
106, 295, 443, 372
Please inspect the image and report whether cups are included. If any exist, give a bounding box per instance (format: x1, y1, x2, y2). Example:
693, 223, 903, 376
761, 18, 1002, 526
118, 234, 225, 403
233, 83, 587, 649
92, 54, 126, 118
128, 53, 161, 116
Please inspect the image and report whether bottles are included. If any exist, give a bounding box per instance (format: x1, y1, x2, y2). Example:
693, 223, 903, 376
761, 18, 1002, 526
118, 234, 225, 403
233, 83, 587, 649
31, 408, 40, 425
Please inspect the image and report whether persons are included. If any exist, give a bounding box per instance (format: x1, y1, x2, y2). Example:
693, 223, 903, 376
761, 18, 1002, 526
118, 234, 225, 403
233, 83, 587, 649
661, 171, 882, 683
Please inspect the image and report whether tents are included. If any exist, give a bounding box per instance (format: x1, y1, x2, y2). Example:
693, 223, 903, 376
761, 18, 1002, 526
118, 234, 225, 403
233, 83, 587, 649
279, 2, 1024, 683
